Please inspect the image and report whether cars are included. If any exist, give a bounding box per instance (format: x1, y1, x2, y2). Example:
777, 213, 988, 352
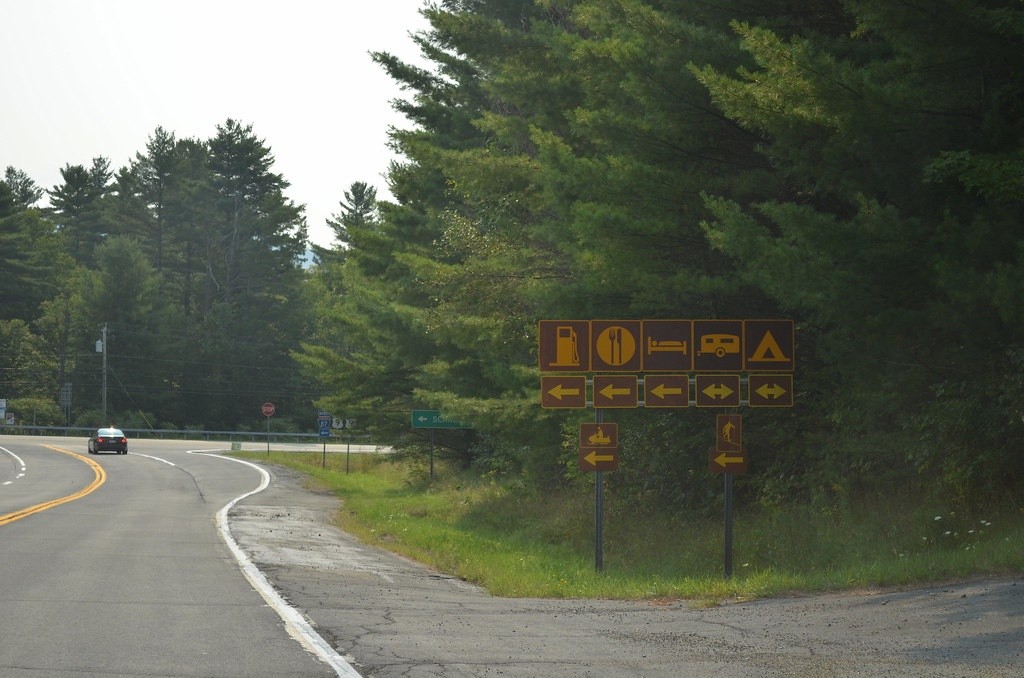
88, 429, 128, 454
